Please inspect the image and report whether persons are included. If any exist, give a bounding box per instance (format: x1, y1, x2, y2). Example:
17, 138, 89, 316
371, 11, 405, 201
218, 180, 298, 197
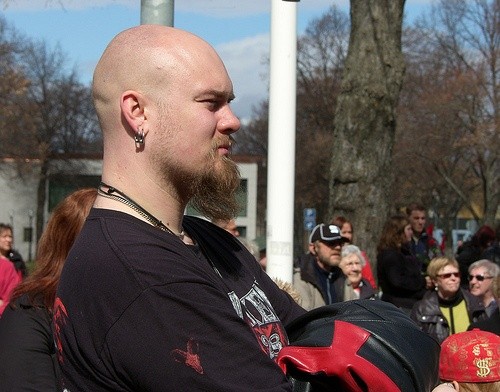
293, 217, 378, 311
376, 203, 437, 314
54, 25, 309, 392
210, 217, 266, 271
0, 189, 99, 392
410, 255, 500, 392
455, 225, 500, 289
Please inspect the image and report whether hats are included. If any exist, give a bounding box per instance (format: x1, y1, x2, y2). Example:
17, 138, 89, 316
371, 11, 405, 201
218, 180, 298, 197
438, 328, 500, 382
309, 223, 352, 243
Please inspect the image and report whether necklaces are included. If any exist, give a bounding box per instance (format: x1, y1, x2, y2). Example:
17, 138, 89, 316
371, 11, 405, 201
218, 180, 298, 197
98, 182, 185, 239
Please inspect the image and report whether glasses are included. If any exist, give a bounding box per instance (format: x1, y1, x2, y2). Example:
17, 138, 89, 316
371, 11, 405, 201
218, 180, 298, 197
437, 272, 460, 279
467, 274, 493, 281
